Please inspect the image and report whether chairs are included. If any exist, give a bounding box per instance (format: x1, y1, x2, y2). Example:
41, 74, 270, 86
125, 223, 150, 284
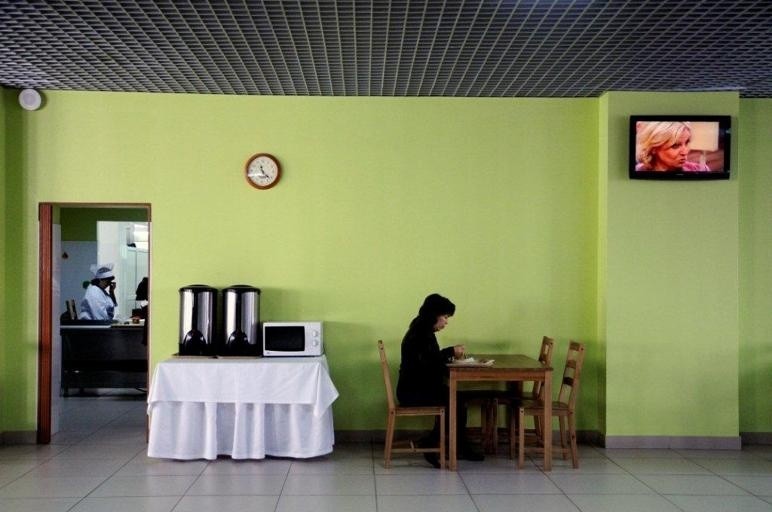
376, 339, 447, 468
487, 336, 556, 459
517, 337, 586, 467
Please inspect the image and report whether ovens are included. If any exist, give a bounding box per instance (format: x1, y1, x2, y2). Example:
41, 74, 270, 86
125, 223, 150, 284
262, 321, 324, 357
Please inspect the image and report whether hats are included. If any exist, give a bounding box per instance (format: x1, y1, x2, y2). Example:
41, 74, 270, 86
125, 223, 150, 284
89, 263, 116, 280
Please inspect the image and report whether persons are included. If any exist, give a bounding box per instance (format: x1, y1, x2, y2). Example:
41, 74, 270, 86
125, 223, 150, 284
77, 266, 118, 322
633, 115, 715, 173
395, 293, 487, 469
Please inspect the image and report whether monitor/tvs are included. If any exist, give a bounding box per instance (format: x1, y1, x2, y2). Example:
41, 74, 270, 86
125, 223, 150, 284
629, 115, 731, 180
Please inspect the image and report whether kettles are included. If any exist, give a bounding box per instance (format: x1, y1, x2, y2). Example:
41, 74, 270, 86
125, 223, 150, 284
178, 284, 219, 355
220, 283, 262, 356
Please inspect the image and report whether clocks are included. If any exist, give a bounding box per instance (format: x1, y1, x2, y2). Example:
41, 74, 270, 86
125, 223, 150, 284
243, 152, 284, 190
